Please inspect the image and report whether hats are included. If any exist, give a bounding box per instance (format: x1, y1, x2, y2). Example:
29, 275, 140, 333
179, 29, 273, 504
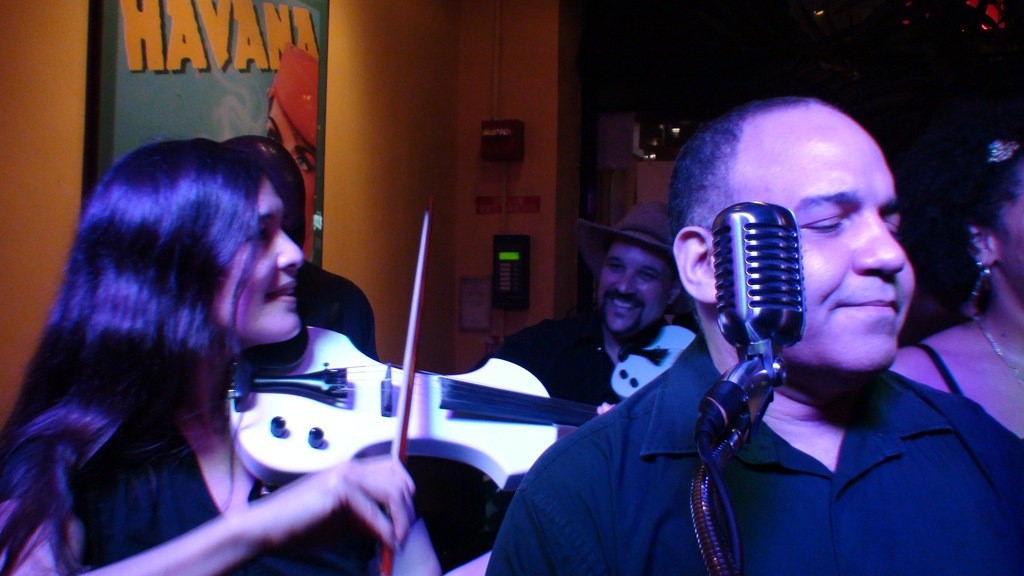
575, 197, 673, 278
269, 43, 319, 148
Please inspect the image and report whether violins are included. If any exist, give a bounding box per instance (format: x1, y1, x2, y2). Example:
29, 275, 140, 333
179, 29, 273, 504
222, 323, 617, 495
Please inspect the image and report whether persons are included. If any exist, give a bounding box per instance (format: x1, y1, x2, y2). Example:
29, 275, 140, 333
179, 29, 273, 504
0, 136, 617, 576
887, 129, 1024, 444
474, 199, 700, 409
219, 137, 377, 362
484, 95, 1024, 576
266, 44, 319, 263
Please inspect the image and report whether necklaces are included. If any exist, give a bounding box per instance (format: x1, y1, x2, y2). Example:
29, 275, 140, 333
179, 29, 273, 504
973, 315, 1024, 388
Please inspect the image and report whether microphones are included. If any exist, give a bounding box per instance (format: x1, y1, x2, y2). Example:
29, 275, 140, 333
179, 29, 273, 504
713, 201, 807, 353
